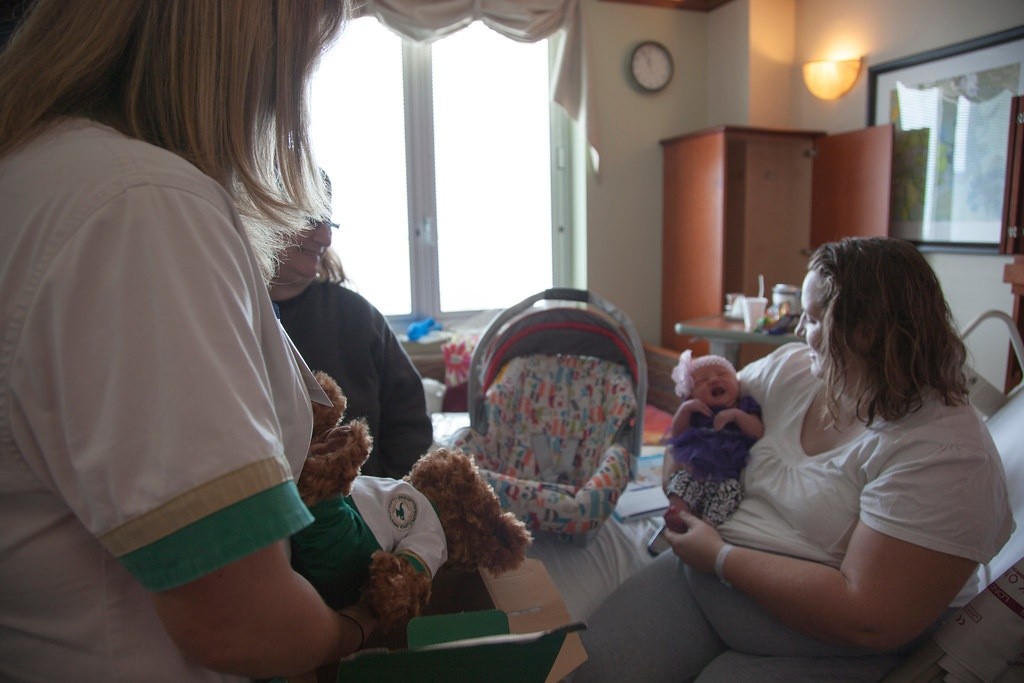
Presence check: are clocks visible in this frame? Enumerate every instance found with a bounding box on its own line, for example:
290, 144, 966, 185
628, 40, 674, 93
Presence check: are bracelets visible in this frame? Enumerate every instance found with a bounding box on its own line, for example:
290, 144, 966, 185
714, 544, 734, 587
341, 609, 370, 648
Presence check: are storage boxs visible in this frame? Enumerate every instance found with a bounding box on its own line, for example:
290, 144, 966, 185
318, 558, 590, 683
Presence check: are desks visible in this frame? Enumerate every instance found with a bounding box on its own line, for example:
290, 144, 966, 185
674, 314, 810, 374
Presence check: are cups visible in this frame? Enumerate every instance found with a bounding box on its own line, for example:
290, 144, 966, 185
772, 284, 801, 313
743, 297, 768, 334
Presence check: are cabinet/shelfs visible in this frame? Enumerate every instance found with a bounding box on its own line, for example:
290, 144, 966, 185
659, 122, 895, 375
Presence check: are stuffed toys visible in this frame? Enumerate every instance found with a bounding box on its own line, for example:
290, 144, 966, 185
290, 368, 536, 629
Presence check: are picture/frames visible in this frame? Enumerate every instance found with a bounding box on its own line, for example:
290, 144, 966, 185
865, 26, 1023, 256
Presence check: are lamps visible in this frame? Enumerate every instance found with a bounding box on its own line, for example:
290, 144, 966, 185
801, 56, 862, 101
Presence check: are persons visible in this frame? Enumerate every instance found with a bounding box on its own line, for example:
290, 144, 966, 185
265, 166, 434, 479
661, 349, 764, 533
0, 0, 372, 683
558, 235, 1017, 683
